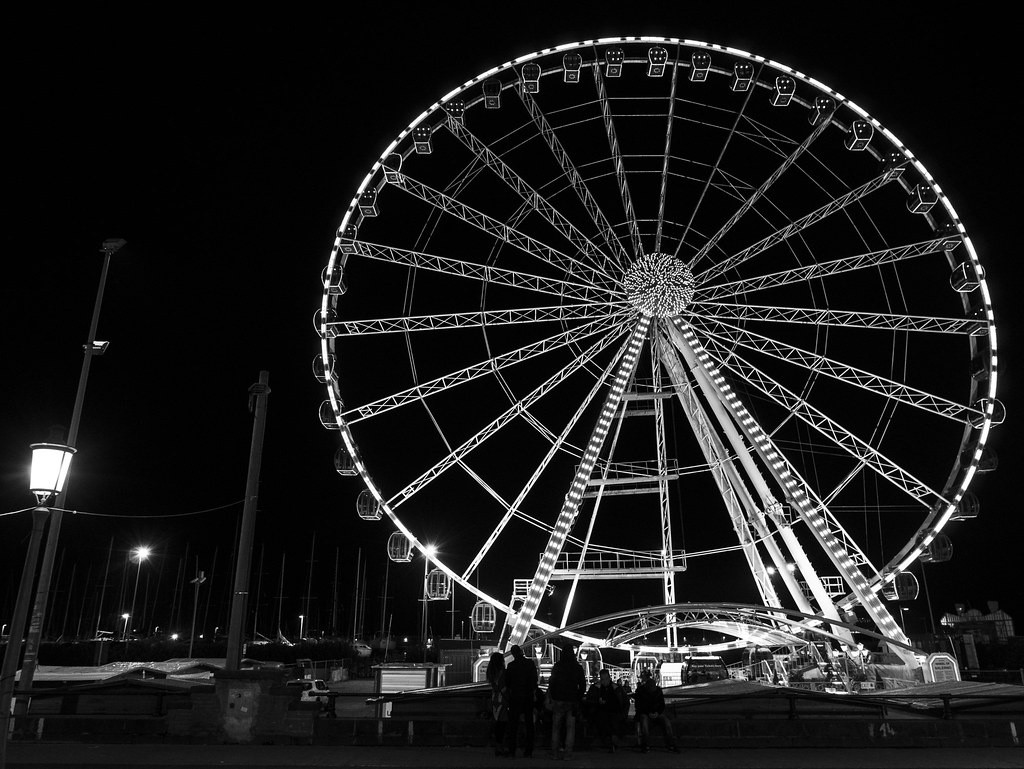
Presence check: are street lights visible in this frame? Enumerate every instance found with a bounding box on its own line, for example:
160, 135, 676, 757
0, 428, 77, 769
225, 382, 272, 670
119, 545, 152, 638
417, 542, 440, 656
189, 571, 207, 657
9, 237, 128, 731
121, 612, 130, 642
899, 607, 908, 636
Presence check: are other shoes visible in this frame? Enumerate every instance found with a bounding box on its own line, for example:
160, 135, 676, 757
495, 751, 504, 756
504, 751, 515, 756
609, 744, 615, 753
640, 746, 654, 754
524, 752, 532, 756
664, 746, 680, 754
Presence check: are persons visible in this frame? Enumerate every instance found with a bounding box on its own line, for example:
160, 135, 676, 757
615, 679, 644, 717
586, 669, 626, 753
549, 644, 586, 761
639, 679, 680, 753
486, 645, 538, 757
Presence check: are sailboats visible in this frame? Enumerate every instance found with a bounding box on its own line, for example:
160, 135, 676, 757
349, 549, 372, 658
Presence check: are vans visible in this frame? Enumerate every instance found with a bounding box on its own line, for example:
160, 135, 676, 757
285, 679, 330, 707
